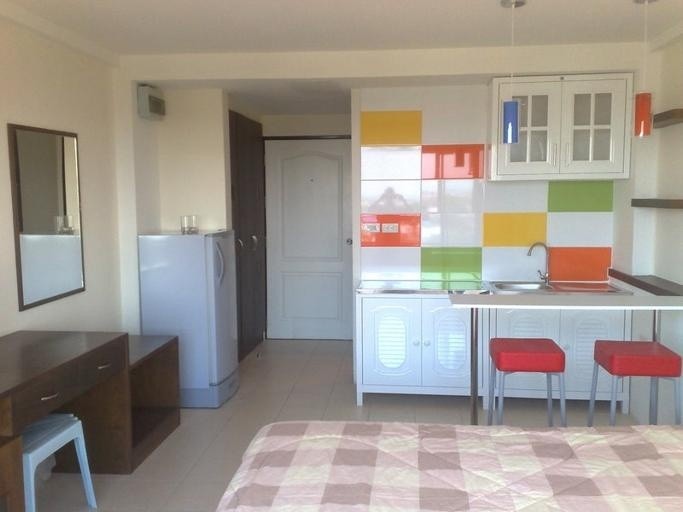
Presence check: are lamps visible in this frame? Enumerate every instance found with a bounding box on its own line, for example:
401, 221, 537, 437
502, 0, 520, 145
632, 1, 652, 138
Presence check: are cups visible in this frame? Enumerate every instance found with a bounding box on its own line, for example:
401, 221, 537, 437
54, 215, 75, 234
181, 215, 197, 236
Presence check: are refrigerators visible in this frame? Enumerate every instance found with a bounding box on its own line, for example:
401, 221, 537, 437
19, 234, 84, 305
137, 229, 241, 408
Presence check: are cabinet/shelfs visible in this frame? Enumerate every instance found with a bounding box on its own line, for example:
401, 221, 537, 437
355, 295, 488, 407
484, 72, 633, 183
490, 309, 632, 414
0, 330, 181, 512
608, 108, 682, 295
229, 112, 266, 362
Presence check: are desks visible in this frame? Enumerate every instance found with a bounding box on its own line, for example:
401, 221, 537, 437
451, 292, 682, 431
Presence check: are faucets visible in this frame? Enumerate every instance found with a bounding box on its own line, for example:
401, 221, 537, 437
528, 242, 549, 284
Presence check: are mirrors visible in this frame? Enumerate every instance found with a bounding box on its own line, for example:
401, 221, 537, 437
6, 124, 86, 313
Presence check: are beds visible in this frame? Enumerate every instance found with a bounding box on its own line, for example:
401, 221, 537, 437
216, 421, 683, 511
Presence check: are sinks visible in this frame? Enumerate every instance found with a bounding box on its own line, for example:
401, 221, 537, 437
488, 281, 556, 293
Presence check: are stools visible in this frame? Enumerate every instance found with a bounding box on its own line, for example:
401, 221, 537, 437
486, 337, 682, 429
21, 413, 97, 512
